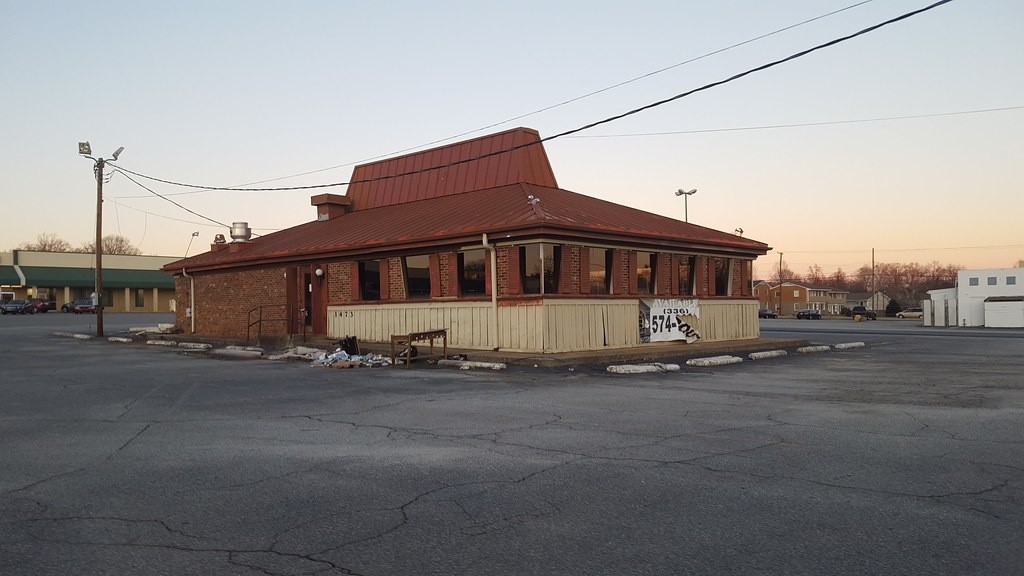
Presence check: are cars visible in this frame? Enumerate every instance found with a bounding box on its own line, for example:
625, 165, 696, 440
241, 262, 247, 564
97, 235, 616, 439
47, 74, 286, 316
75, 299, 103, 314
797, 309, 822, 320
758, 308, 778, 320
61, 300, 86, 313
0, 301, 8, 315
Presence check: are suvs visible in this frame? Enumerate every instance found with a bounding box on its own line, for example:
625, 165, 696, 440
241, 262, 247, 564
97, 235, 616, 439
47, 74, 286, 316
3, 300, 32, 315
852, 306, 877, 321
29, 298, 51, 313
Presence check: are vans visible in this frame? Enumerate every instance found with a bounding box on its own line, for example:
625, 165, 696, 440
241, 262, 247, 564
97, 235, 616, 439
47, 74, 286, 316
896, 307, 925, 319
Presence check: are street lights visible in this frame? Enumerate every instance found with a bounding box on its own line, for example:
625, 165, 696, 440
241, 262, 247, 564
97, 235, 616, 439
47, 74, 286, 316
675, 189, 698, 223
777, 251, 784, 315
78, 141, 124, 337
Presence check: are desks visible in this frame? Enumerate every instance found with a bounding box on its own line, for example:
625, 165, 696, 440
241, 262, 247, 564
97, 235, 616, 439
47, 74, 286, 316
391, 328, 449, 368
